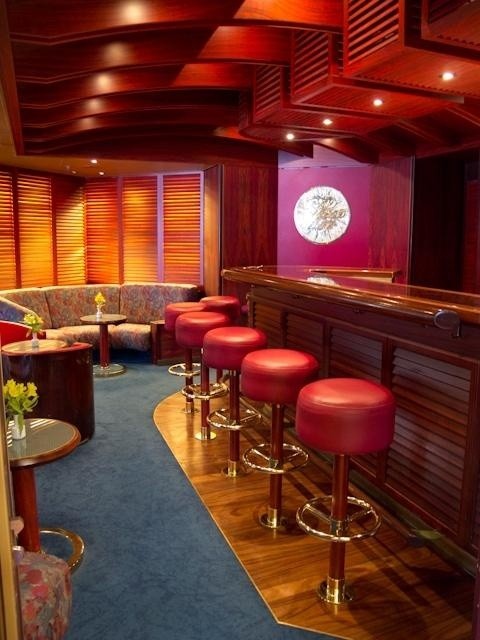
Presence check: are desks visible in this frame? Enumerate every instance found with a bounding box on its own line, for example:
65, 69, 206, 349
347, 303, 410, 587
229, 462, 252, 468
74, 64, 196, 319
80, 314, 128, 377
7, 418, 84, 574
0, 339, 93, 445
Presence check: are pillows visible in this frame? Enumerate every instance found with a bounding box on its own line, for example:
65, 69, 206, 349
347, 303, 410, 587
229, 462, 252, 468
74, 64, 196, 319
0, 283, 198, 329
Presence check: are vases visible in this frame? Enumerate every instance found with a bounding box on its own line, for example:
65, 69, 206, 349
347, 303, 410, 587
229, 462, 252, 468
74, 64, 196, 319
11, 415, 26, 440
31, 332, 39, 347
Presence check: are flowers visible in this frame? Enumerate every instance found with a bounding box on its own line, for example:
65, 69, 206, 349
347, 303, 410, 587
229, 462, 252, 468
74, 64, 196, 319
3, 379, 39, 436
18, 313, 44, 338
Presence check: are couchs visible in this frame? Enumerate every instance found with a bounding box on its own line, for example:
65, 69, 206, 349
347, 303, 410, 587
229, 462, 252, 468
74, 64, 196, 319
0, 283, 206, 366
